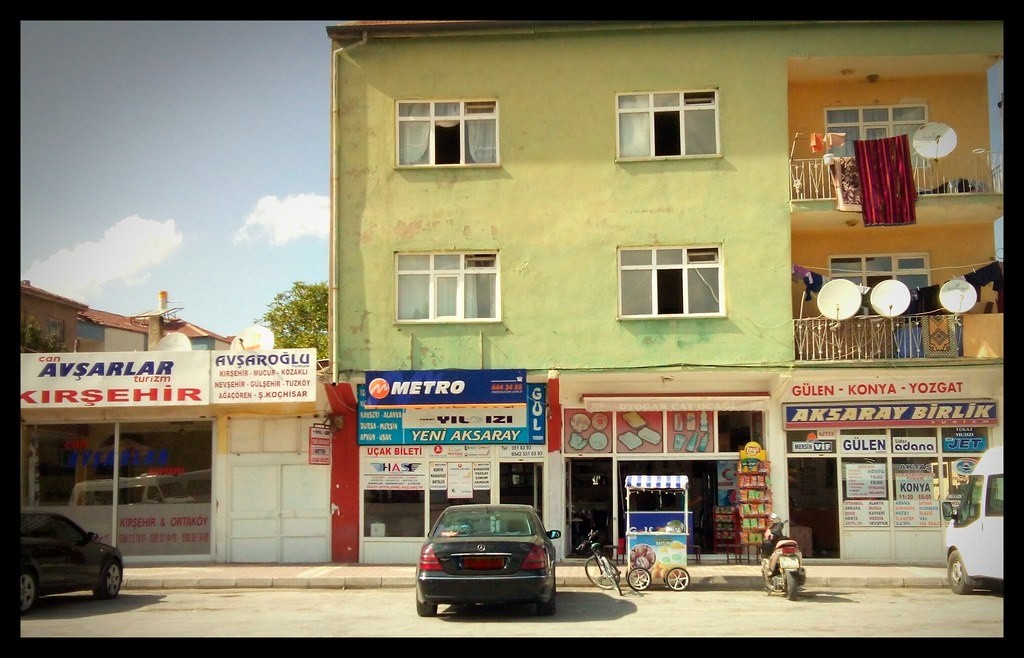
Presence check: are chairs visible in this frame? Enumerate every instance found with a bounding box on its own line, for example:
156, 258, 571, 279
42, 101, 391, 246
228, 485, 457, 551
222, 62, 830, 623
457, 519, 478, 534
725, 528, 750, 564
507, 519, 526, 534
687, 528, 701, 564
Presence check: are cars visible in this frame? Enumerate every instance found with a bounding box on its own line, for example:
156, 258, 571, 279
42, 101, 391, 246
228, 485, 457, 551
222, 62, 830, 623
415, 503, 561, 617
21, 508, 124, 617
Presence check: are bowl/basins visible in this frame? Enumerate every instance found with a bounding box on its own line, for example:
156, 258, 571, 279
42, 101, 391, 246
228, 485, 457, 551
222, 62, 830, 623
629, 544, 657, 572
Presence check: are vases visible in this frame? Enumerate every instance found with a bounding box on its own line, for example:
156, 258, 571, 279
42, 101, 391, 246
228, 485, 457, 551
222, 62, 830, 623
823, 153, 834, 166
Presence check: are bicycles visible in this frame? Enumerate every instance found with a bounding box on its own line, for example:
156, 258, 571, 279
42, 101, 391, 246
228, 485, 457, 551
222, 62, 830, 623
576, 529, 624, 598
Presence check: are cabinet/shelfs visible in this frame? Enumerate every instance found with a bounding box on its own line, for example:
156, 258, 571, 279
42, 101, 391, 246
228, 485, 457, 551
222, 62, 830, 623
712, 507, 739, 554
738, 471, 769, 548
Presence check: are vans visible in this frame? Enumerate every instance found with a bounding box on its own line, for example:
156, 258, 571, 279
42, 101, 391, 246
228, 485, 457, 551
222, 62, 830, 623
178, 469, 212, 503
942, 444, 1004, 594
68, 472, 197, 506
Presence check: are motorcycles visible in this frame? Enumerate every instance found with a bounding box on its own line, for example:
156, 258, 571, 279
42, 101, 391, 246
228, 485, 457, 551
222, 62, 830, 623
756, 512, 807, 601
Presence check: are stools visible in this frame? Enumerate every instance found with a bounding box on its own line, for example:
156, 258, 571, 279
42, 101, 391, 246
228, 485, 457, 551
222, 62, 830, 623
741, 543, 759, 565
604, 545, 624, 566
